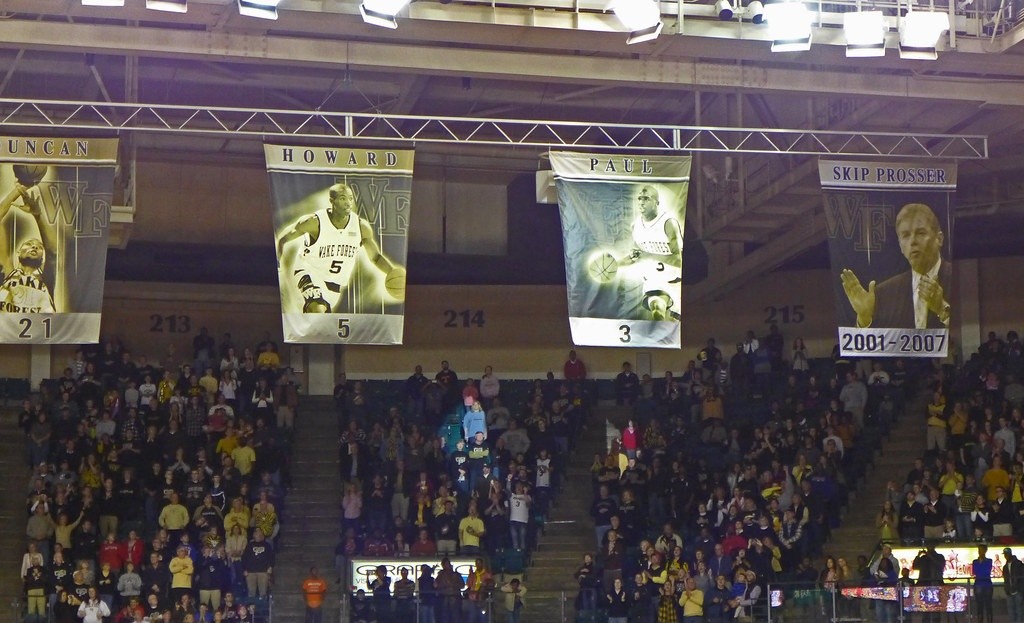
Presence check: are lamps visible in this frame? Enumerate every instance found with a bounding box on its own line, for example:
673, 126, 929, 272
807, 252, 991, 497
81, 0, 951, 62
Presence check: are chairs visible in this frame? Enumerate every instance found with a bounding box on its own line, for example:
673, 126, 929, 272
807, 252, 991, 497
344, 375, 689, 428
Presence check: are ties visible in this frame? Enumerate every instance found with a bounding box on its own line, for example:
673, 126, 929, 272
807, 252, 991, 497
916, 275, 927, 329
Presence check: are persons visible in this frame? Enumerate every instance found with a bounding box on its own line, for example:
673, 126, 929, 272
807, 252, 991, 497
1003, 549, 1024, 623
304, 567, 327, 623
971, 544, 994, 622
840, 203, 950, 329
587, 186, 682, 321
334, 350, 588, 621
18, 327, 302, 623
276, 185, 405, 315
0, 163, 60, 311
577, 325, 946, 623
939, 331, 1024, 546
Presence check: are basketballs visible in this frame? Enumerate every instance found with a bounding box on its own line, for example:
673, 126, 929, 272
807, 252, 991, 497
587, 252, 617, 282
12, 165, 48, 185
385, 269, 405, 300
